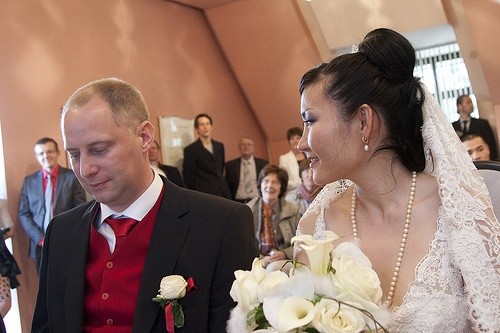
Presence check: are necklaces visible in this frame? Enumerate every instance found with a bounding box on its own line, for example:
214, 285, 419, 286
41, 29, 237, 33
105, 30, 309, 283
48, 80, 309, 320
350, 168, 417, 310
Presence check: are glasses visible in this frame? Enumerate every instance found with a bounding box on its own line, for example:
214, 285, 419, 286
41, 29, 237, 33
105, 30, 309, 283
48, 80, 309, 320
261, 178, 281, 186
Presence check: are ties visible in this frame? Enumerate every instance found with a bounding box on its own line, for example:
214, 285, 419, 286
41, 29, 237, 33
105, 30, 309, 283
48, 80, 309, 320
104, 217, 139, 250
463, 120, 467, 134
244, 161, 253, 195
43, 174, 53, 237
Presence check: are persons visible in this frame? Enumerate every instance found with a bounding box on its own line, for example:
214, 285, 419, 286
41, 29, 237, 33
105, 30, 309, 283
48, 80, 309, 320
227, 138, 270, 204
225, 28, 500, 333
278, 127, 307, 194
451, 94, 496, 162
0, 226, 21, 333
182, 113, 232, 200
246, 165, 303, 276
148, 141, 186, 189
285, 158, 326, 218
30, 78, 260, 333
18, 137, 87, 278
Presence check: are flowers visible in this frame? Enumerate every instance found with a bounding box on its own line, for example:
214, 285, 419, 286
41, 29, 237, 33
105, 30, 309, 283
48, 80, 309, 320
152, 274, 197, 333
229, 212, 383, 333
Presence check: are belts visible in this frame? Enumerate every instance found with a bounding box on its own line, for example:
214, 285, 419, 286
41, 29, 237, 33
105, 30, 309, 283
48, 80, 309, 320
234, 200, 250, 203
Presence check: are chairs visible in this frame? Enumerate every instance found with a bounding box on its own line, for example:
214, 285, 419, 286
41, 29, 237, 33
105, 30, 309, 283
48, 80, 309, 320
472, 160, 500, 225
